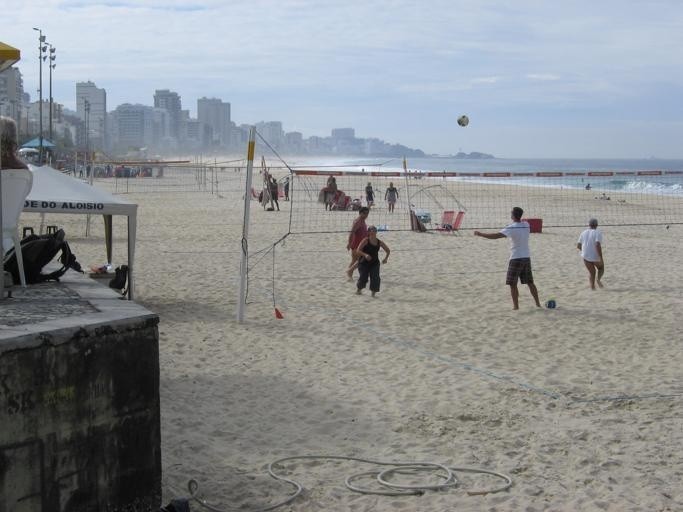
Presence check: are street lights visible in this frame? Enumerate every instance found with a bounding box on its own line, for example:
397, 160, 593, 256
81, 96, 91, 152
45, 42, 56, 162
32, 26, 46, 166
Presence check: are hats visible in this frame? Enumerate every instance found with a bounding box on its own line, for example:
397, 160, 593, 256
589, 219, 598, 225
368, 226, 377, 231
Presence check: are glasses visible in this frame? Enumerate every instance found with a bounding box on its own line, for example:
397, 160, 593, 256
363, 211, 369, 214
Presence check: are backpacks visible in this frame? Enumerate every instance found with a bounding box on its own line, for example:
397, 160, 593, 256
109, 266, 130, 296
4, 229, 73, 283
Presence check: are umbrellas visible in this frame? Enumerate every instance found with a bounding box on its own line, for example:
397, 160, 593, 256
21, 137, 57, 147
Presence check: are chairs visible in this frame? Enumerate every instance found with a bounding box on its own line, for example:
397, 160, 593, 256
0, 169, 33, 299
415, 208, 464, 237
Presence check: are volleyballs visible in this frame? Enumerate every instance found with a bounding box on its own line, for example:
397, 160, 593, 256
545, 297, 558, 308
457, 115, 469, 126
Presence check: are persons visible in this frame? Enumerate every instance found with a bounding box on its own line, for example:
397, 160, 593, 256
355, 225, 391, 297
474, 208, 541, 310
251, 174, 290, 211
0, 116, 28, 168
326, 177, 337, 211
366, 182, 375, 210
346, 206, 370, 281
15, 147, 148, 179
576, 219, 605, 290
344, 199, 361, 210
384, 181, 399, 213
327, 174, 334, 185
